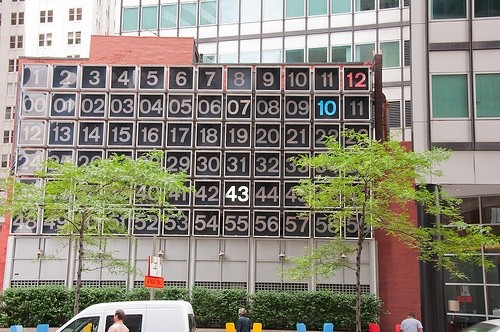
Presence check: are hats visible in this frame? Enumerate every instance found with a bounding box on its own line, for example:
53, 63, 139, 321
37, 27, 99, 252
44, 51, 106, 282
238, 308, 247, 315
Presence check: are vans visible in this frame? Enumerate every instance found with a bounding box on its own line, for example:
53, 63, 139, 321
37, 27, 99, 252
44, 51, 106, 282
53, 298, 197, 332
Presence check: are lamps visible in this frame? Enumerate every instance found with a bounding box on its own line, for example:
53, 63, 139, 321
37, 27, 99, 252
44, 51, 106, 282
158, 250, 165, 256
340, 252, 346, 259
99, 250, 104, 254
219, 251, 225, 256
36, 249, 43, 256
448, 300, 459, 324
279, 251, 285, 258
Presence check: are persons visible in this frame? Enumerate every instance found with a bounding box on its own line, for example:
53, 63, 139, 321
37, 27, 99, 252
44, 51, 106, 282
400, 313, 423, 332
236, 308, 250, 332
108, 309, 129, 332
